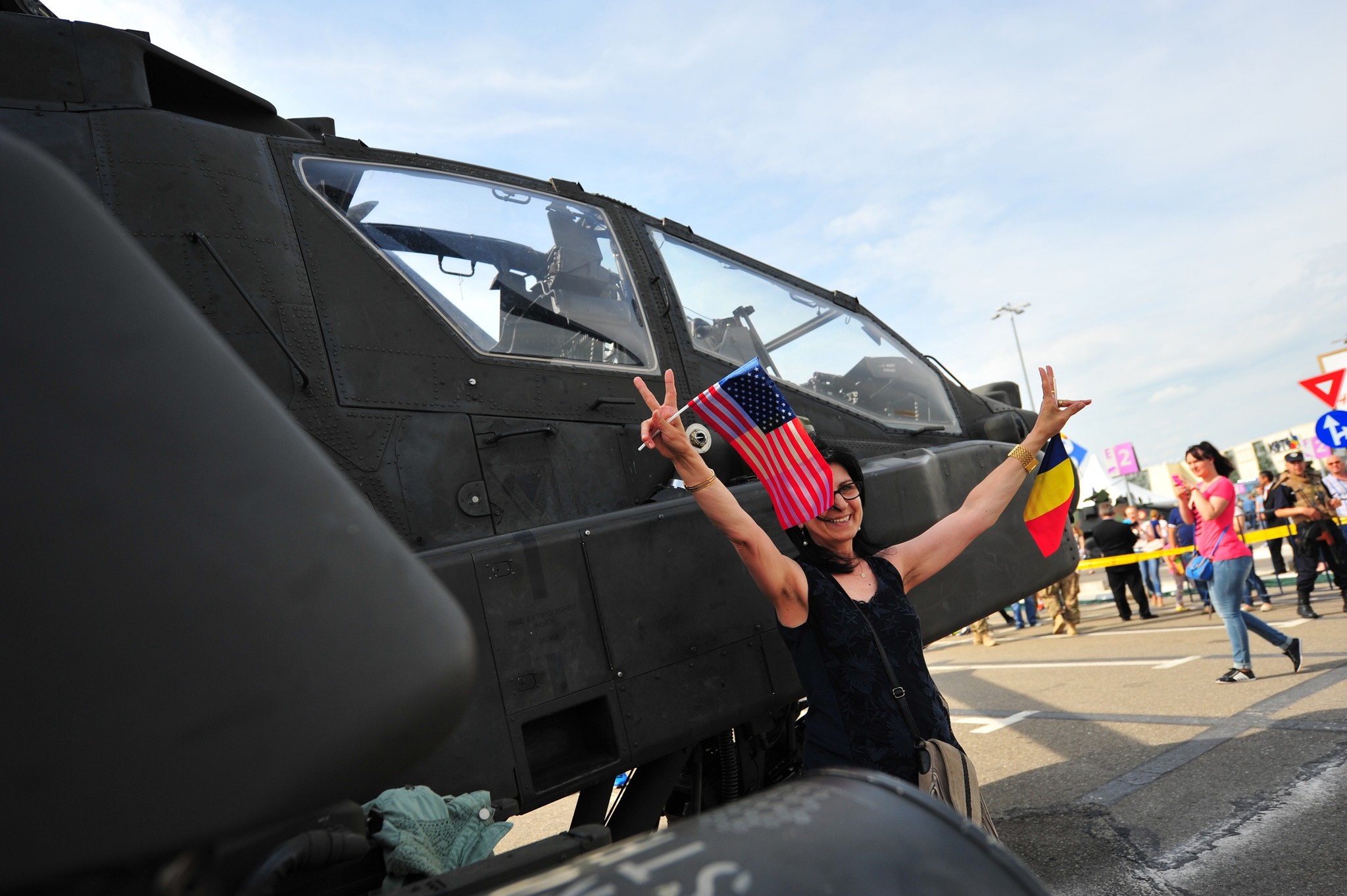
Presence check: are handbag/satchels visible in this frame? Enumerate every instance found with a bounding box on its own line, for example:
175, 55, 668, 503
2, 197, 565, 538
917, 738, 982, 830
1185, 556, 1213, 581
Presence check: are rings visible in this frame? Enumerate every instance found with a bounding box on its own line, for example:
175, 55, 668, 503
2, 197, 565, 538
1051, 390, 1055, 393
1058, 400, 1062, 407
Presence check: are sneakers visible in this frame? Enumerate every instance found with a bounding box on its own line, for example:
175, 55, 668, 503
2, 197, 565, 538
1282, 638, 1303, 672
1216, 668, 1257, 684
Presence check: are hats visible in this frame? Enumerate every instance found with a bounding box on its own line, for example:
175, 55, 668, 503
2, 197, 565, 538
1284, 452, 1303, 462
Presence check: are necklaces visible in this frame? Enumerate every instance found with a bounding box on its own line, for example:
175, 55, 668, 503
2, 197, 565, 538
847, 559, 866, 578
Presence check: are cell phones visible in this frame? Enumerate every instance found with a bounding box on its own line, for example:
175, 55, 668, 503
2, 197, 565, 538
1172, 474, 1185, 488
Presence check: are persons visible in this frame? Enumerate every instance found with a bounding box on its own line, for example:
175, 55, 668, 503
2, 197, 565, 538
630, 366, 1091, 822
1072, 502, 1272, 621
1174, 442, 1303, 684
1233, 452, 1347, 619
949, 568, 1082, 648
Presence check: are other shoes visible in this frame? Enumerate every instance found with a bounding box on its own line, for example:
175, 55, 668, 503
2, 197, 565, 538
1176, 605, 1190, 612
1052, 614, 1067, 634
973, 632, 997, 647
1122, 616, 1130, 620
1141, 610, 1158, 619
1297, 604, 1317, 617
1260, 603, 1273, 611
1202, 606, 1216, 614
1240, 603, 1256, 611
1066, 623, 1078, 634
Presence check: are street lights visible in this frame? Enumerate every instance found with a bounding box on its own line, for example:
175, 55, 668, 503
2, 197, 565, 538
992, 301, 1037, 413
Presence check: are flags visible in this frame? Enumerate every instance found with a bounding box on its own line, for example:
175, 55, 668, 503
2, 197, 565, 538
688, 358, 834, 531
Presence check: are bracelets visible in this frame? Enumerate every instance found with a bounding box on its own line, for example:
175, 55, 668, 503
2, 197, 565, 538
682, 468, 715, 493
1009, 443, 1039, 474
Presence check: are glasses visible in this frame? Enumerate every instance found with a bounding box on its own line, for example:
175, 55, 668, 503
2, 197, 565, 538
1329, 461, 1340, 465
834, 481, 862, 500
